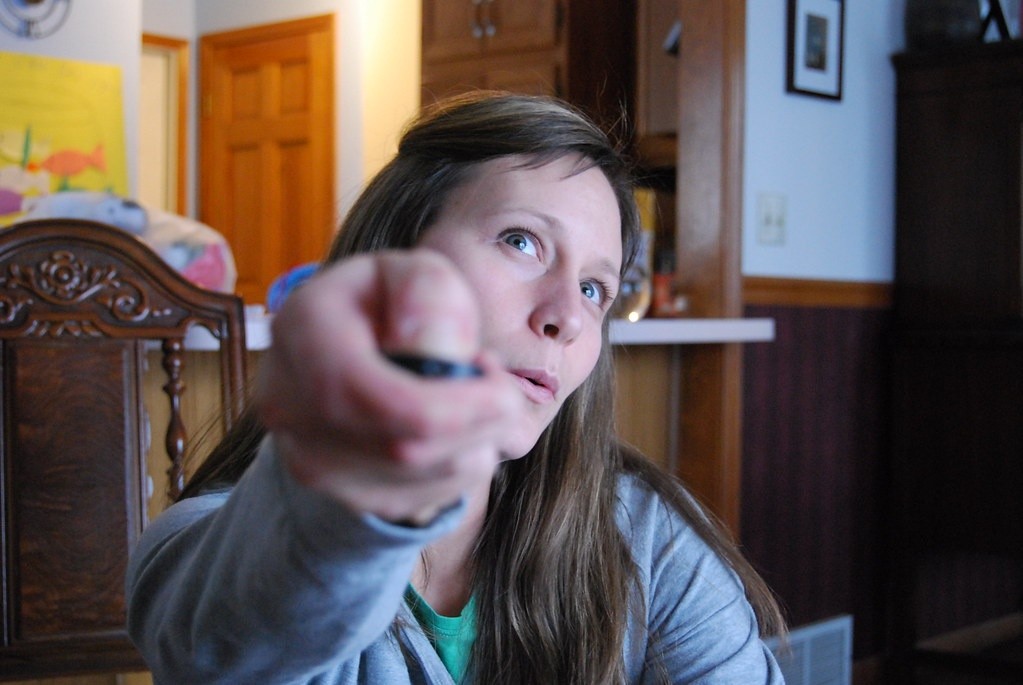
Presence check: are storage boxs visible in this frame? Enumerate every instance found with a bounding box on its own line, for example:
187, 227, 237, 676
617, 186, 676, 320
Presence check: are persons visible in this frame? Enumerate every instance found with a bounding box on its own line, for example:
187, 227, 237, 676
124, 92, 793, 685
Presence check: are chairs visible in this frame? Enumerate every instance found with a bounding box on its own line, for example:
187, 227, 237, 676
1, 215, 244, 684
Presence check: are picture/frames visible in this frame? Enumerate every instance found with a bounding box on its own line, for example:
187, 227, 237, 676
783, 0, 847, 100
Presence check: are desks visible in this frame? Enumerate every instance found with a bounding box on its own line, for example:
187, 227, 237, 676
0, 314, 780, 684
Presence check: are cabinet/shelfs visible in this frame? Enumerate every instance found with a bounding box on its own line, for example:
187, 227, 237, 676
420, 0, 682, 174
